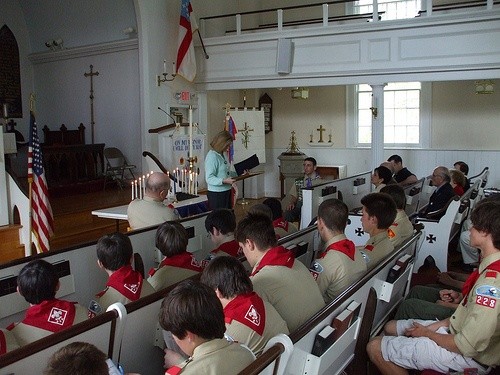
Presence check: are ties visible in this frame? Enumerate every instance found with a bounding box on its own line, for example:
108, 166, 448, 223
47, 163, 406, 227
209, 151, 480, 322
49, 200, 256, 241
461, 260, 500, 304
306, 179, 312, 187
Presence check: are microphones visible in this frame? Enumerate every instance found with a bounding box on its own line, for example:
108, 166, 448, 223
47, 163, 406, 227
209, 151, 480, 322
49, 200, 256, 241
158, 107, 176, 125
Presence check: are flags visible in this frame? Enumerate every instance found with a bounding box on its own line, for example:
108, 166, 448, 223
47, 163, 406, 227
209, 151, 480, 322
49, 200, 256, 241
25, 110, 56, 254
176, 0, 200, 82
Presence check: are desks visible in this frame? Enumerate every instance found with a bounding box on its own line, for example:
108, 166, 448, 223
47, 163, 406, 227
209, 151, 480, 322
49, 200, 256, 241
92, 195, 207, 234
316, 165, 348, 179
39, 143, 108, 180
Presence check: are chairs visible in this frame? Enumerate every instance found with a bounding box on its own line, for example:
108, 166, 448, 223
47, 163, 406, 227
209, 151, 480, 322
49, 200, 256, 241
104, 148, 137, 190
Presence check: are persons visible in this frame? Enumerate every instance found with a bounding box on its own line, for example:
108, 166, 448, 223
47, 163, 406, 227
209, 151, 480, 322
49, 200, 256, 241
204, 130, 248, 212
285, 157, 322, 222
126, 172, 182, 232
0, 154, 500, 375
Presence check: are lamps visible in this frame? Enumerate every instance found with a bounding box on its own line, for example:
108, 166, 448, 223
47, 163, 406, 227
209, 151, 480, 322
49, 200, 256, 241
291, 87, 310, 100
46, 38, 64, 50
157, 61, 176, 86
475, 80, 496, 95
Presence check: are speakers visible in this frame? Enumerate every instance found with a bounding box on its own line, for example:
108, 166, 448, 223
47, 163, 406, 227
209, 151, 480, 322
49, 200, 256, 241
276, 37, 291, 74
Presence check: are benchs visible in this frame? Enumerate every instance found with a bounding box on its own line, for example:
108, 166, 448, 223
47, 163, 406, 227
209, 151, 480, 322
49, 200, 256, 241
343, 179, 425, 247
298, 170, 372, 230
0, 210, 423, 375
412, 167, 490, 276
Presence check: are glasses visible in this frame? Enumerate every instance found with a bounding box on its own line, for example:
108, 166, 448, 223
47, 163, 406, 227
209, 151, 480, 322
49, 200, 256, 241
433, 173, 441, 177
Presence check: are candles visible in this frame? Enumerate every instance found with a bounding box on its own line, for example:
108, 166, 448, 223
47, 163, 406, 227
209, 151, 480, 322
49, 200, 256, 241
5, 103, 8, 118
132, 169, 198, 201
